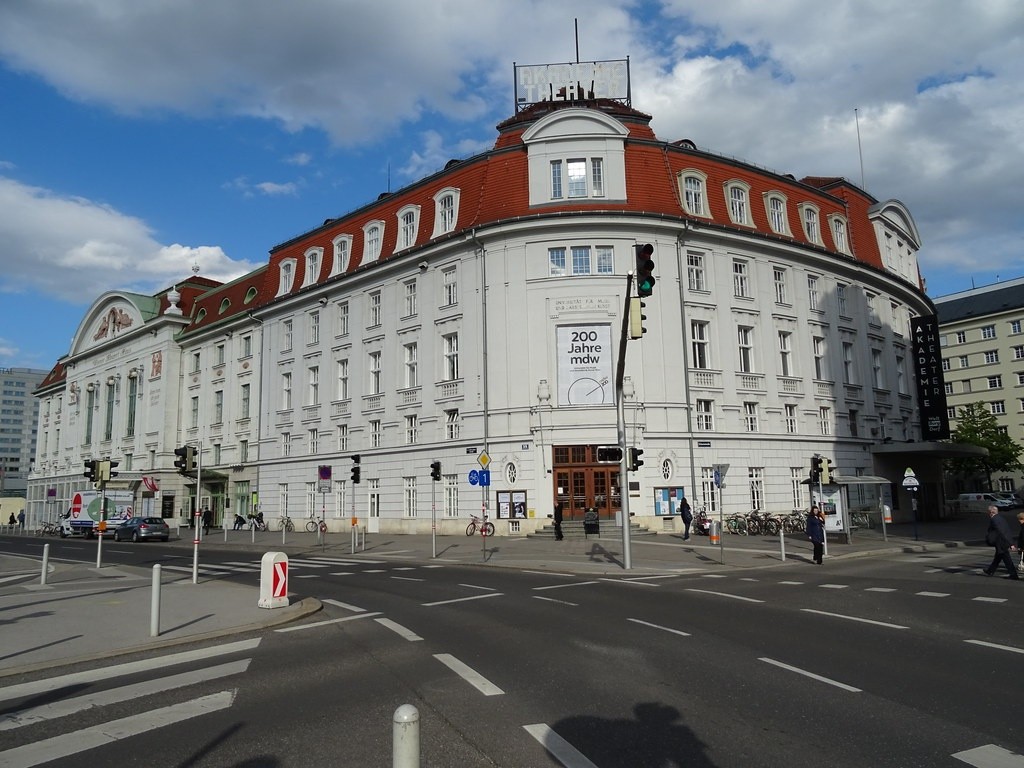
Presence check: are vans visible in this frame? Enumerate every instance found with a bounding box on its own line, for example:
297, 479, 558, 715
958, 492, 1014, 511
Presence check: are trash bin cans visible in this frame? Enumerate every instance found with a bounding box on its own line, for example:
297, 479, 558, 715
884, 505, 892, 523
710, 520, 720, 545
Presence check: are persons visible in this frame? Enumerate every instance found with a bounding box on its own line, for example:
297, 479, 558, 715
806, 506, 825, 565
553, 499, 563, 541
680, 497, 692, 542
232, 514, 246, 530
17, 510, 25, 528
202, 507, 212, 535
8, 513, 17, 529
1017, 512, 1024, 570
983, 506, 1018, 579
514, 503, 525, 517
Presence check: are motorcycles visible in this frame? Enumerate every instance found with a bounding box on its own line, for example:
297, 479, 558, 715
694, 511, 712, 536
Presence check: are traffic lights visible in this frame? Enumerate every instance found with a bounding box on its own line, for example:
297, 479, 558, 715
596, 446, 623, 463
628, 448, 644, 471
351, 454, 360, 463
629, 297, 647, 340
635, 243, 656, 297
811, 458, 834, 483
84, 461, 119, 482
430, 462, 441, 480
173, 447, 197, 470
351, 466, 360, 483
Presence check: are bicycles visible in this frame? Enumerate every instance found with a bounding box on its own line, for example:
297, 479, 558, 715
466, 514, 495, 537
726, 509, 874, 536
35, 520, 61, 537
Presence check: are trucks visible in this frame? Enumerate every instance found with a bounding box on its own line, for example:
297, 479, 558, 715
59, 490, 134, 539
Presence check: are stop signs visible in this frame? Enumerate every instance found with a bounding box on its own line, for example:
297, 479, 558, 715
72, 494, 82, 518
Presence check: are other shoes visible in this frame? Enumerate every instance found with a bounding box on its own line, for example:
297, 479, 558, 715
685, 538, 690, 541
821, 563, 824, 565
983, 568, 995, 575
1008, 574, 1018, 579
812, 556, 817, 563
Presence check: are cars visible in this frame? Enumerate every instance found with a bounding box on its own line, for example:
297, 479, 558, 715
114, 516, 170, 543
999, 492, 1023, 508
